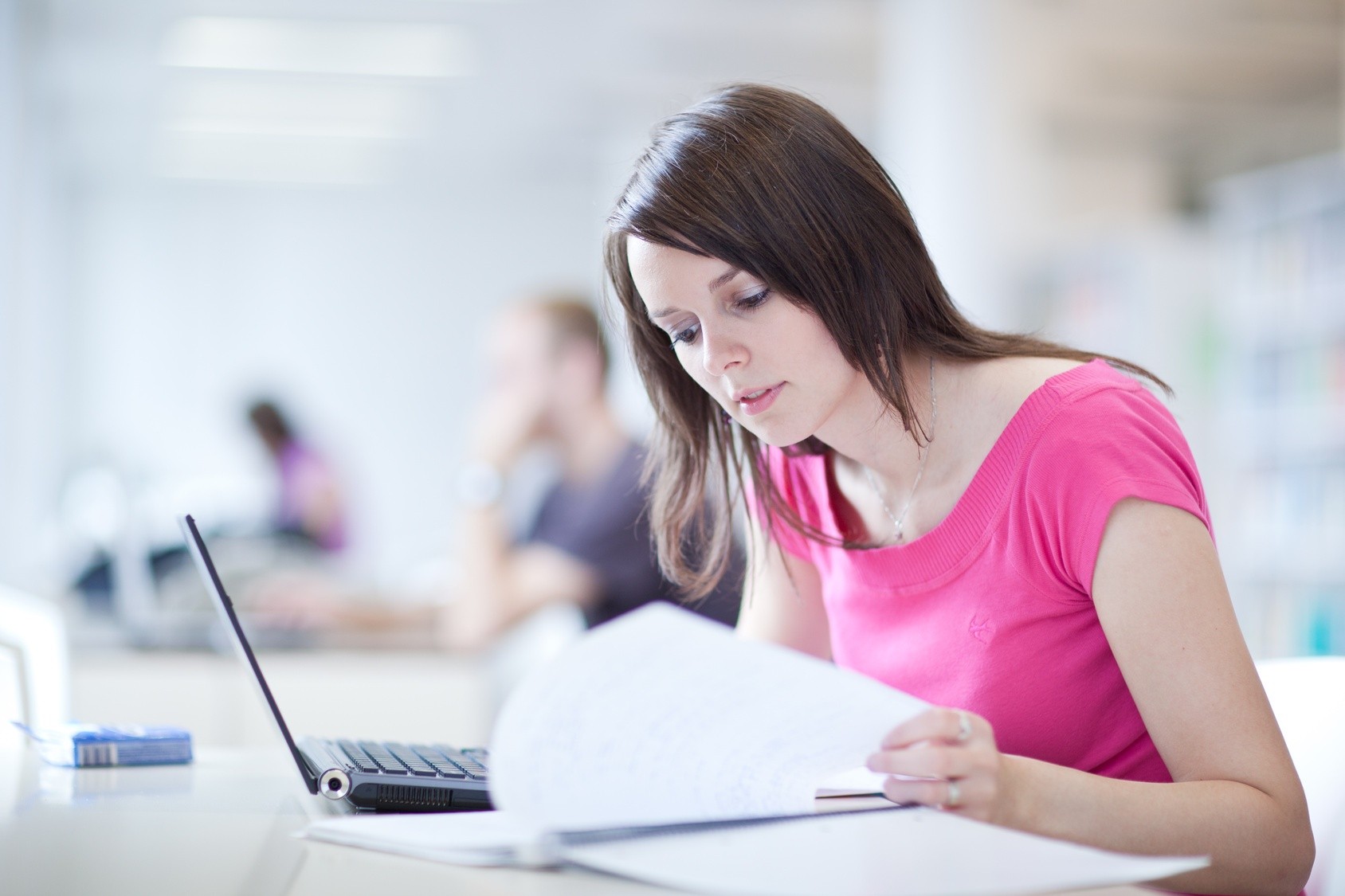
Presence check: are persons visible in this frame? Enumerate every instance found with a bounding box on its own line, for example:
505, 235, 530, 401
251, 400, 352, 556
446, 286, 747, 654
604, 83, 1316, 895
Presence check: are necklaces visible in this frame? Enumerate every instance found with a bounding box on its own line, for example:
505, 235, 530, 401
861, 352, 932, 540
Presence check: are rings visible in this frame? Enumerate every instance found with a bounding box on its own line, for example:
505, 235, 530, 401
950, 708, 972, 743
944, 783, 963, 809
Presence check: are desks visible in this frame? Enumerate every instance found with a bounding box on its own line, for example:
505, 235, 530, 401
0, 657, 1170, 896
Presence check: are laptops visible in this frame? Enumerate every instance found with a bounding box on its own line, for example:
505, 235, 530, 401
178, 513, 494, 815
